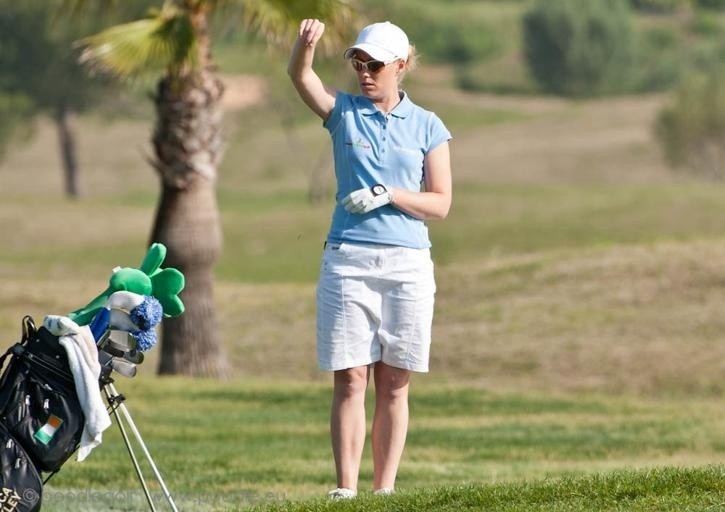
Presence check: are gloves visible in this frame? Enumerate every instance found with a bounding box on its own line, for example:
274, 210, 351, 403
340, 184, 391, 214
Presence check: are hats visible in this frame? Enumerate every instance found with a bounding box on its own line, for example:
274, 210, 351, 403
345, 21, 410, 62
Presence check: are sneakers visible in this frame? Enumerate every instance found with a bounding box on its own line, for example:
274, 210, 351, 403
370, 489, 395, 497
327, 488, 356, 502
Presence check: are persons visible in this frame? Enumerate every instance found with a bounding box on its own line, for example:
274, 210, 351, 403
283, 16, 453, 503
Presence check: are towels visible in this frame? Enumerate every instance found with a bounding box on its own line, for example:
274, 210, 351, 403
58, 324, 112, 463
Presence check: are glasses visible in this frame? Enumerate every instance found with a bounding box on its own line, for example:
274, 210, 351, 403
350, 56, 386, 70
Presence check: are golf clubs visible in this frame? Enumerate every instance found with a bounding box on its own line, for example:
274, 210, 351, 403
97, 330, 144, 378
